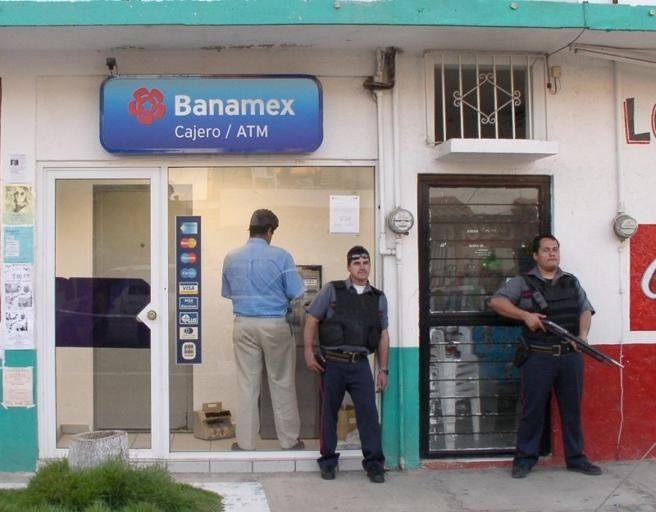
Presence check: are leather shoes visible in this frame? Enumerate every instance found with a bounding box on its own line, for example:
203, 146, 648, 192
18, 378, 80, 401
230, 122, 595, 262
367, 469, 385, 484
567, 461, 602, 476
321, 466, 336, 481
510, 462, 533, 478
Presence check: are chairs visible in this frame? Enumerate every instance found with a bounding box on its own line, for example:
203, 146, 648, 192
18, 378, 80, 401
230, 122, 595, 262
429, 326, 482, 450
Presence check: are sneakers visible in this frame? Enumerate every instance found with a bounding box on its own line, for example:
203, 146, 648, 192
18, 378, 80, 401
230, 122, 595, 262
281, 439, 305, 450
231, 442, 257, 451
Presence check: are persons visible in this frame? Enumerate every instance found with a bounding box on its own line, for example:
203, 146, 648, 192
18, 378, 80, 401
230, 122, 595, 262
487, 234, 603, 478
222, 208, 307, 451
474, 298, 523, 451
303, 245, 391, 481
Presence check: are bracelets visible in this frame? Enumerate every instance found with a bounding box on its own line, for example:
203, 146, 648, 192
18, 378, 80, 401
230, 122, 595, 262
379, 366, 389, 375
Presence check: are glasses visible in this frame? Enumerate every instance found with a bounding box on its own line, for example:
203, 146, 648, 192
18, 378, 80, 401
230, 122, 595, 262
351, 252, 370, 259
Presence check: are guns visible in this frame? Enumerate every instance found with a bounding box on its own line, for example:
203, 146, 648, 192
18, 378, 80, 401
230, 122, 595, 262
541, 320, 626, 370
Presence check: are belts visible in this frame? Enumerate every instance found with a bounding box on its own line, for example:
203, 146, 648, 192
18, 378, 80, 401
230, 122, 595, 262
323, 349, 368, 364
528, 342, 574, 357
